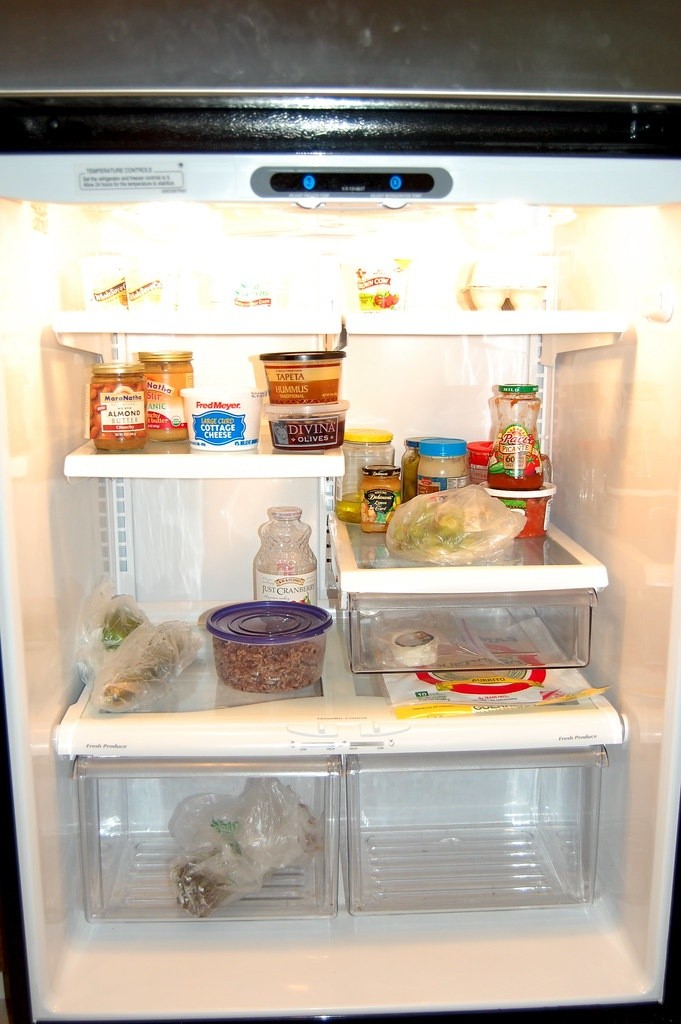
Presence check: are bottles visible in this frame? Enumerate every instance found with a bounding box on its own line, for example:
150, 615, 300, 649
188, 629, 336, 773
336, 431, 396, 523
253, 508, 318, 611
487, 383, 545, 488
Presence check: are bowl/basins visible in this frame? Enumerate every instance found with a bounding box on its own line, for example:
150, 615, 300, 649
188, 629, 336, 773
479, 482, 556, 539
206, 601, 334, 693
261, 399, 349, 451
179, 387, 267, 451
256, 351, 348, 409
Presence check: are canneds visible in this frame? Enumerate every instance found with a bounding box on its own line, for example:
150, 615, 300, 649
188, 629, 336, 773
89, 351, 194, 451
360, 465, 401, 533
401, 437, 469, 502
487, 383, 545, 489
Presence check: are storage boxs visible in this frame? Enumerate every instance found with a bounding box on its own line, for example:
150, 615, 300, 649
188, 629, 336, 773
327, 525, 599, 674
74, 744, 341, 923
340, 743, 611, 918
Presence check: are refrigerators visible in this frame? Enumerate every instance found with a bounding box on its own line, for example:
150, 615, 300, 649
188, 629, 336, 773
0, 0, 681, 1024
